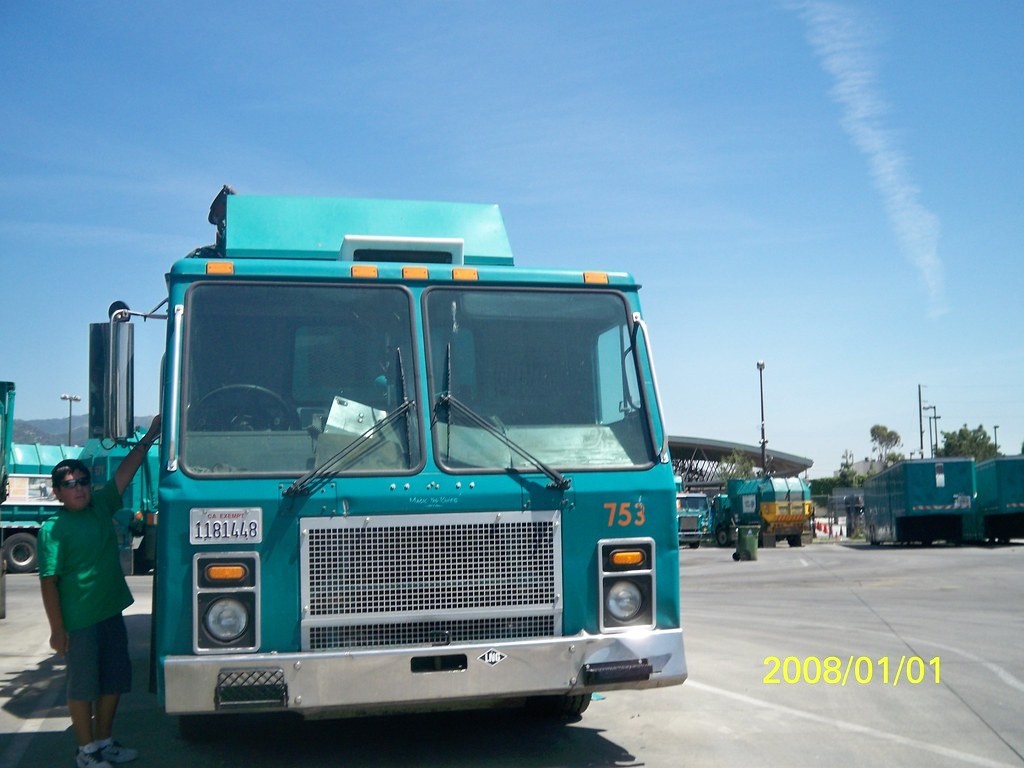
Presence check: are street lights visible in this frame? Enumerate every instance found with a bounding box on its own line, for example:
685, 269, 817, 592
928, 416, 941, 459
59, 393, 82, 445
922, 404, 938, 457
993, 424, 1000, 456
756, 360, 769, 476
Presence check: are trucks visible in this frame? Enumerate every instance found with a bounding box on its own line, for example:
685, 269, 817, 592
710, 471, 815, 549
1, 182, 815, 740
858, 452, 1023, 549
673, 475, 712, 551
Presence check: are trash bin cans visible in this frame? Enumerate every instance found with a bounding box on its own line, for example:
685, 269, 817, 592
730, 525, 762, 562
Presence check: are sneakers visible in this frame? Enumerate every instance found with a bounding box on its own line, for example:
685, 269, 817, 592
74, 748, 112, 768
98, 741, 139, 762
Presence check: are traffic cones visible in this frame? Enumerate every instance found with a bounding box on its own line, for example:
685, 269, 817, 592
815, 521, 840, 541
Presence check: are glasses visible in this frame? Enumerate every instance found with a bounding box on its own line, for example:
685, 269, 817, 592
60, 476, 90, 489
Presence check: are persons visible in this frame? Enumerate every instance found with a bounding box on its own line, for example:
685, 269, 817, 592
39, 413, 162, 768
